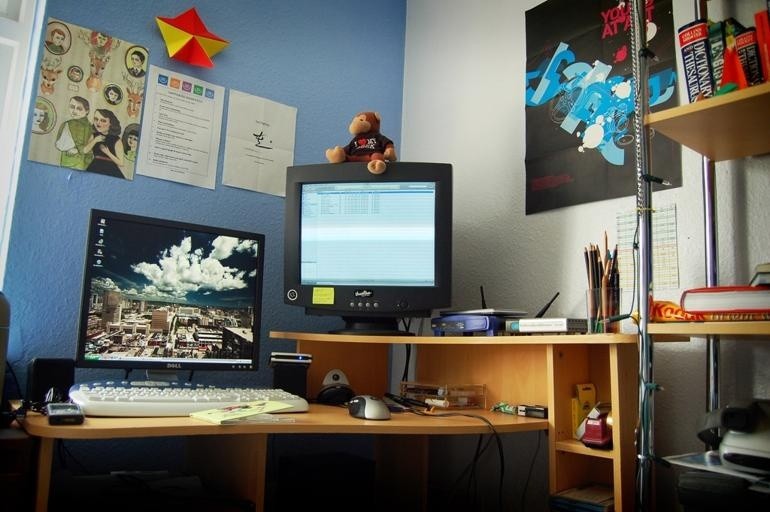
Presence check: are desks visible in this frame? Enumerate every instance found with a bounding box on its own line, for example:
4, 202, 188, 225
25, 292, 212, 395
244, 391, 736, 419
7, 335, 690, 511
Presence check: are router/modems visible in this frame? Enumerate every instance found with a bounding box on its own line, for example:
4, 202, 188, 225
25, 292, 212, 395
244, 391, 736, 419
267, 352, 312, 368
505, 317, 587, 334
431, 285, 559, 337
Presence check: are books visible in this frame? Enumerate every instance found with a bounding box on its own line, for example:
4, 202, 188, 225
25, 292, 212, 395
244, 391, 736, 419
677, 11, 770, 105
680, 261, 770, 315
190, 400, 297, 426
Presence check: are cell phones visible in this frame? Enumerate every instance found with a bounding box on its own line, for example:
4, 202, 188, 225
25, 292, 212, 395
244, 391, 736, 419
47, 403, 83, 425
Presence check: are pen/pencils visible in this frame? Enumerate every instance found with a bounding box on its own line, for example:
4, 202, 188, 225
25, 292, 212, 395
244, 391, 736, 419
581, 231, 621, 333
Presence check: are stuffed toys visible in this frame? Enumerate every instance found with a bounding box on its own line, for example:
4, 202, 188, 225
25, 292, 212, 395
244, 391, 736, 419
324, 111, 398, 176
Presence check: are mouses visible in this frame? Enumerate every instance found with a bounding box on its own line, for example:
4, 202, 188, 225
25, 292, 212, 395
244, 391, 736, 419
348, 394, 391, 421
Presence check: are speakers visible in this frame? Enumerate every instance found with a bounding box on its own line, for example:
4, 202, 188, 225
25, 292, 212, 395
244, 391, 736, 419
26, 358, 75, 402
273, 366, 306, 398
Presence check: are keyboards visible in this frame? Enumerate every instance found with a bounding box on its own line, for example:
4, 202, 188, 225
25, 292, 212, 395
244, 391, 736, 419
69, 379, 310, 418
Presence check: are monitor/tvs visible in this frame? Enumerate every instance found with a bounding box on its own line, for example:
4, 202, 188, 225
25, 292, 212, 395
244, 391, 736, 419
74, 207, 265, 371
283, 162, 452, 335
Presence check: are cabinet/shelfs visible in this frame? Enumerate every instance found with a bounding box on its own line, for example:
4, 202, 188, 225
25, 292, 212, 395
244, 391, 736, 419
631, 0, 769, 512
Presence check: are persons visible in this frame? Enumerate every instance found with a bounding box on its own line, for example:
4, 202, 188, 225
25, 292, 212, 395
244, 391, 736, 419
83, 109, 125, 178
95, 31, 110, 49
29, 103, 53, 133
126, 50, 145, 75
105, 86, 122, 103
47, 30, 66, 53
56, 96, 92, 170
126, 130, 136, 162
